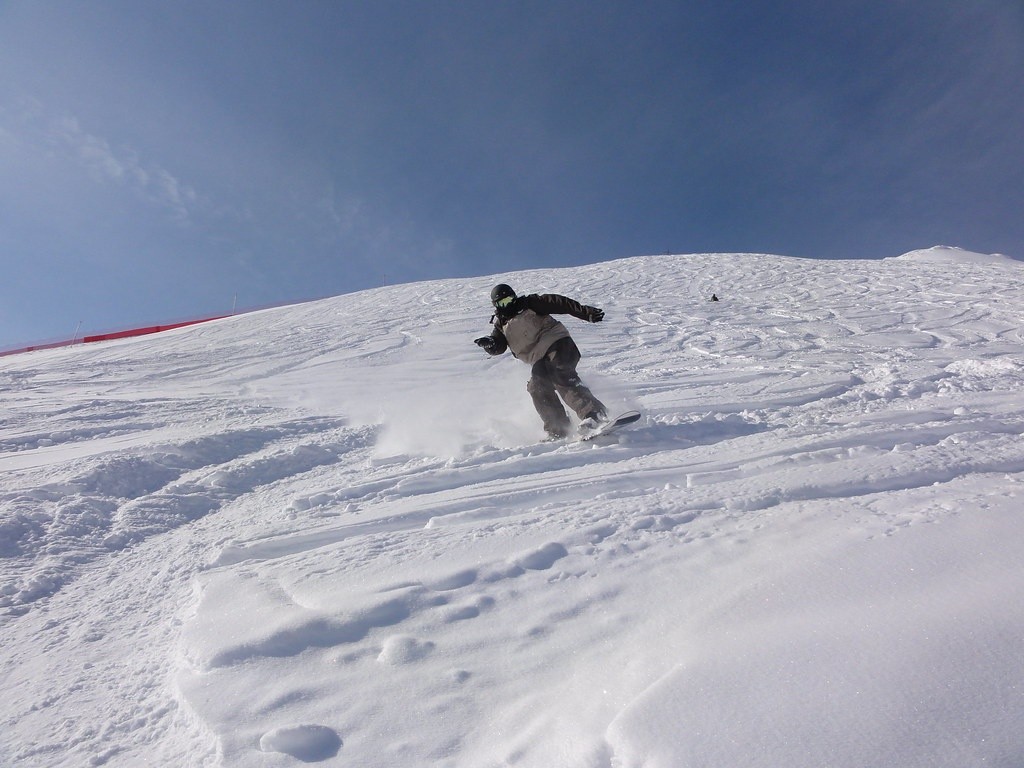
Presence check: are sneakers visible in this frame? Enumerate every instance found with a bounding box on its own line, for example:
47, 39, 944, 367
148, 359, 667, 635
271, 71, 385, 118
545, 425, 571, 438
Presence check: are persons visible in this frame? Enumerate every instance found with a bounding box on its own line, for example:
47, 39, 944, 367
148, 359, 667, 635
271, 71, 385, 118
474, 284, 606, 441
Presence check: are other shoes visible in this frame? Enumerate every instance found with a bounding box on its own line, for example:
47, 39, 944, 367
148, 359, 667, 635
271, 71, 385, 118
577, 406, 609, 435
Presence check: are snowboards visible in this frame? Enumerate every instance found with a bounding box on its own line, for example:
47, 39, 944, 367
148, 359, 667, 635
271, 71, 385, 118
536, 409, 643, 443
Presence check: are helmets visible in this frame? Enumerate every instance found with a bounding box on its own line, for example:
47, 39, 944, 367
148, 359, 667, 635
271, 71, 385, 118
491, 284, 516, 307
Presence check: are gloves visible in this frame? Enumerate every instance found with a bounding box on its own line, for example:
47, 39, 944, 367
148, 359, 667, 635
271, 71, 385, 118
586, 306, 605, 324
475, 336, 495, 350
